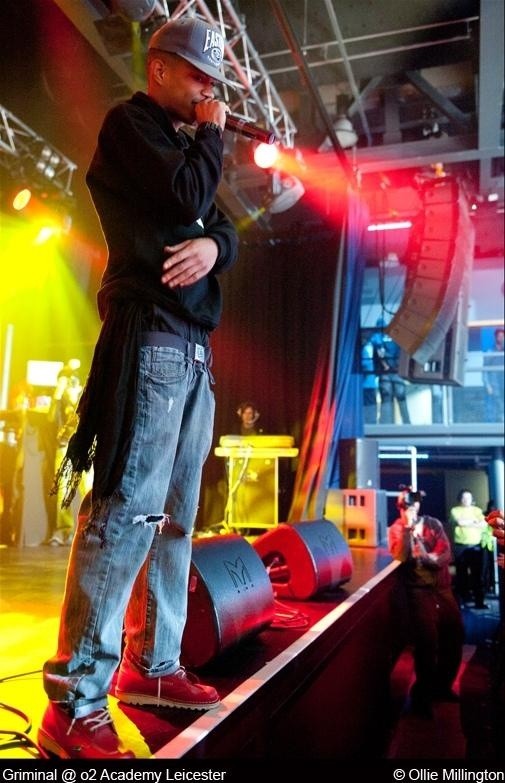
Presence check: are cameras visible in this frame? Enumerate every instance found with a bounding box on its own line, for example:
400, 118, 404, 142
398, 483, 426, 511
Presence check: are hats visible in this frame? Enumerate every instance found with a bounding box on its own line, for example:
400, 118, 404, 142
145, 16, 240, 92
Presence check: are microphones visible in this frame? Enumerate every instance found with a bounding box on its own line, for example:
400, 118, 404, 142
224, 112, 276, 145
248, 417, 258, 424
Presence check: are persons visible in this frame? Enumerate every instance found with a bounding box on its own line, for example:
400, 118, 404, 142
493, 327, 505, 351
489, 508, 505, 546
230, 400, 267, 434
37, 16, 278, 758
450, 488, 489, 609
496, 552, 505, 570
481, 499, 496, 592
388, 486, 465, 719
45, 366, 94, 546
373, 331, 412, 423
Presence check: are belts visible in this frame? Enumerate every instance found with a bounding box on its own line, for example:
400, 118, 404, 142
136, 327, 213, 368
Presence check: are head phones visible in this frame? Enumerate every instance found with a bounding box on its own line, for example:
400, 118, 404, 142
236, 401, 260, 417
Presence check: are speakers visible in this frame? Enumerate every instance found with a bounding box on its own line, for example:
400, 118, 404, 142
251, 519, 353, 601
384, 174, 476, 389
179, 532, 276, 668
340, 437, 381, 489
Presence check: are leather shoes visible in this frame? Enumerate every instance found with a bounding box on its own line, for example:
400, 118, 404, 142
35, 693, 136, 759
114, 656, 221, 711
